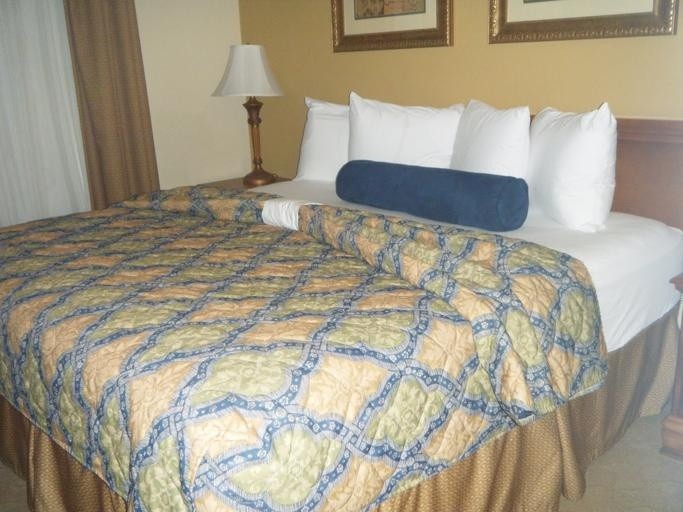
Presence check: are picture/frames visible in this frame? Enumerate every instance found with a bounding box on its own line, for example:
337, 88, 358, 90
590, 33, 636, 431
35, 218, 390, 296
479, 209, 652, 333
331, 1, 453, 52
488, 0, 679, 44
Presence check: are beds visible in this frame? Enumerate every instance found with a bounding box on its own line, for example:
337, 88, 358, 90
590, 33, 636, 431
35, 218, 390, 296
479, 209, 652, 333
1, 117, 683, 510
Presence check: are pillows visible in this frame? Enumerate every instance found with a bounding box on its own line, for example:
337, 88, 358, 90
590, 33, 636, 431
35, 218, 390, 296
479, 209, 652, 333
335, 160, 529, 232
448, 97, 530, 184
347, 90, 464, 169
291, 97, 349, 182
530, 101, 618, 231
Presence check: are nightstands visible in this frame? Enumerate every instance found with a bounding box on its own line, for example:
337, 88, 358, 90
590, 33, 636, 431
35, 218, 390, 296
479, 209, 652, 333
660, 276, 682, 459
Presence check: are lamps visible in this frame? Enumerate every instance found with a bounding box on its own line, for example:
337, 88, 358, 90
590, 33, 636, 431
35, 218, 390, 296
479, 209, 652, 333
209, 44, 285, 187
